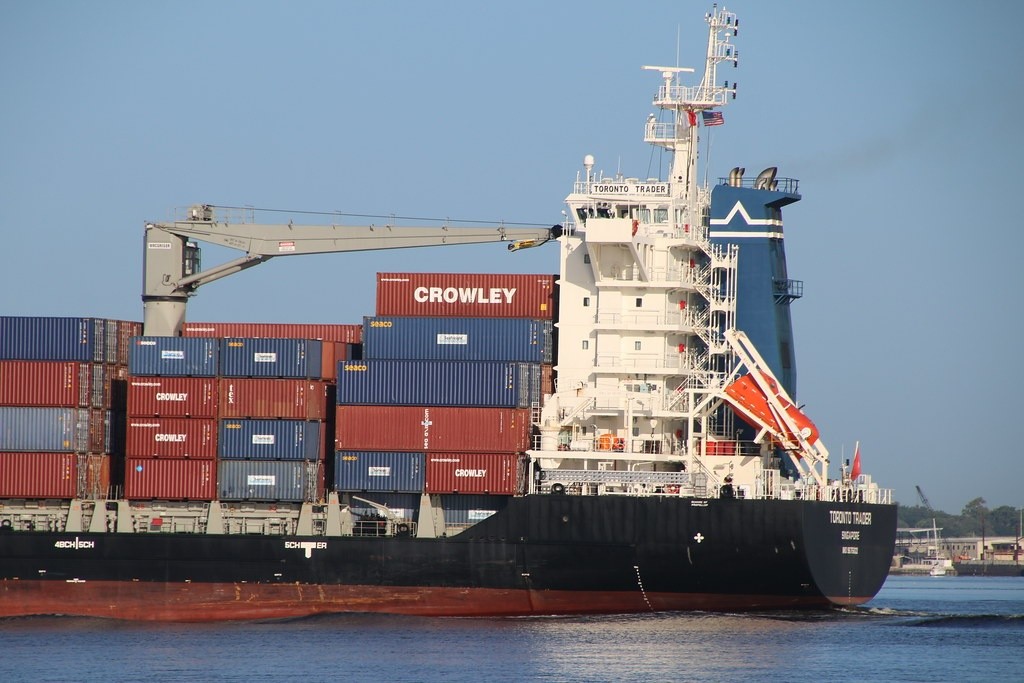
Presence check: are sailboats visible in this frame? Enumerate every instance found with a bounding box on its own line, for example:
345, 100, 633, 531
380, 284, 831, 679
928, 519, 946, 577
952, 500, 1024, 577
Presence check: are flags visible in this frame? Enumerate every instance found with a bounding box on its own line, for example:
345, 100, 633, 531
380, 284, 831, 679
850, 441, 862, 482
703, 111, 724, 126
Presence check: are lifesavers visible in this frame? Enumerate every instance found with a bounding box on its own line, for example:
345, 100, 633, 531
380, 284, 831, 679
550, 482, 566, 496
719, 492, 732, 503
396, 523, 410, 536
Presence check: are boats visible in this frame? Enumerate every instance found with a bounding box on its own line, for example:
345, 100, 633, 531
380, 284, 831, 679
0, 0, 901, 627
